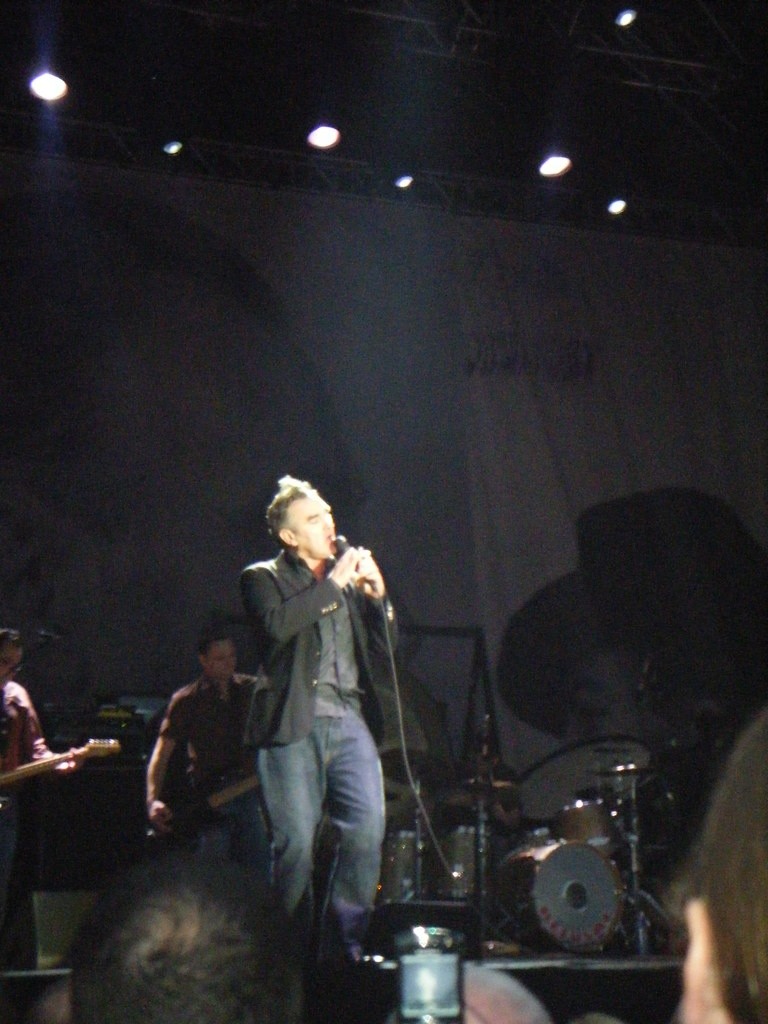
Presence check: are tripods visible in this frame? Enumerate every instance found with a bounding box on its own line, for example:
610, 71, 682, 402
613, 778, 674, 957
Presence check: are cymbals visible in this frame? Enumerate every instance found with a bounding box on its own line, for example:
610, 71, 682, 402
514, 738, 651, 820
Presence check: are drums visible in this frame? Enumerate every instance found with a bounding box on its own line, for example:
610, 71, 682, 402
548, 797, 621, 855
375, 831, 423, 902
494, 840, 628, 953
429, 825, 504, 905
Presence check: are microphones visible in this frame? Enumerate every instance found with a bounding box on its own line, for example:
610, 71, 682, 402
334, 536, 379, 593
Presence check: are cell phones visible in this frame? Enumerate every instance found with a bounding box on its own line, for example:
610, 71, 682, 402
399, 929, 465, 1024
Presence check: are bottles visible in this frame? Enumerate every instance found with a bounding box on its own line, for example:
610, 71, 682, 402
634, 913, 648, 954
394, 930, 462, 1024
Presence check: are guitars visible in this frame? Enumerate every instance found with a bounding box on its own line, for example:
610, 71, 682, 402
127, 768, 263, 857
0, 738, 120, 811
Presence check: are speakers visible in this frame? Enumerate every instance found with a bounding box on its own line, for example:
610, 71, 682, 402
11, 891, 96, 968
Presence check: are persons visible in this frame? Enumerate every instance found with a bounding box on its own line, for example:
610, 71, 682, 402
670, 710, 768, 1024
430, 740, 520, 861
32, 859, 305, 1023
0, 628, 87, 929
238, 474, 404, 988
146, 626, 271, 888
